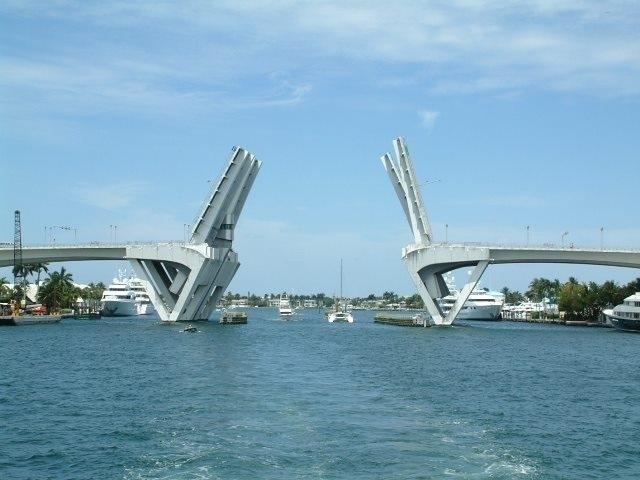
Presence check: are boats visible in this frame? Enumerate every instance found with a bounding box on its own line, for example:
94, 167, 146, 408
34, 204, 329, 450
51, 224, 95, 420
0, 315, 62, 326
100, 268, 154, 316
184, 328, 197, 332
439, 269, 505, 320
326, 299, 434, 327
601, 292, 640, 332
218, 311, 248, 324
279, 300, 294, 315
